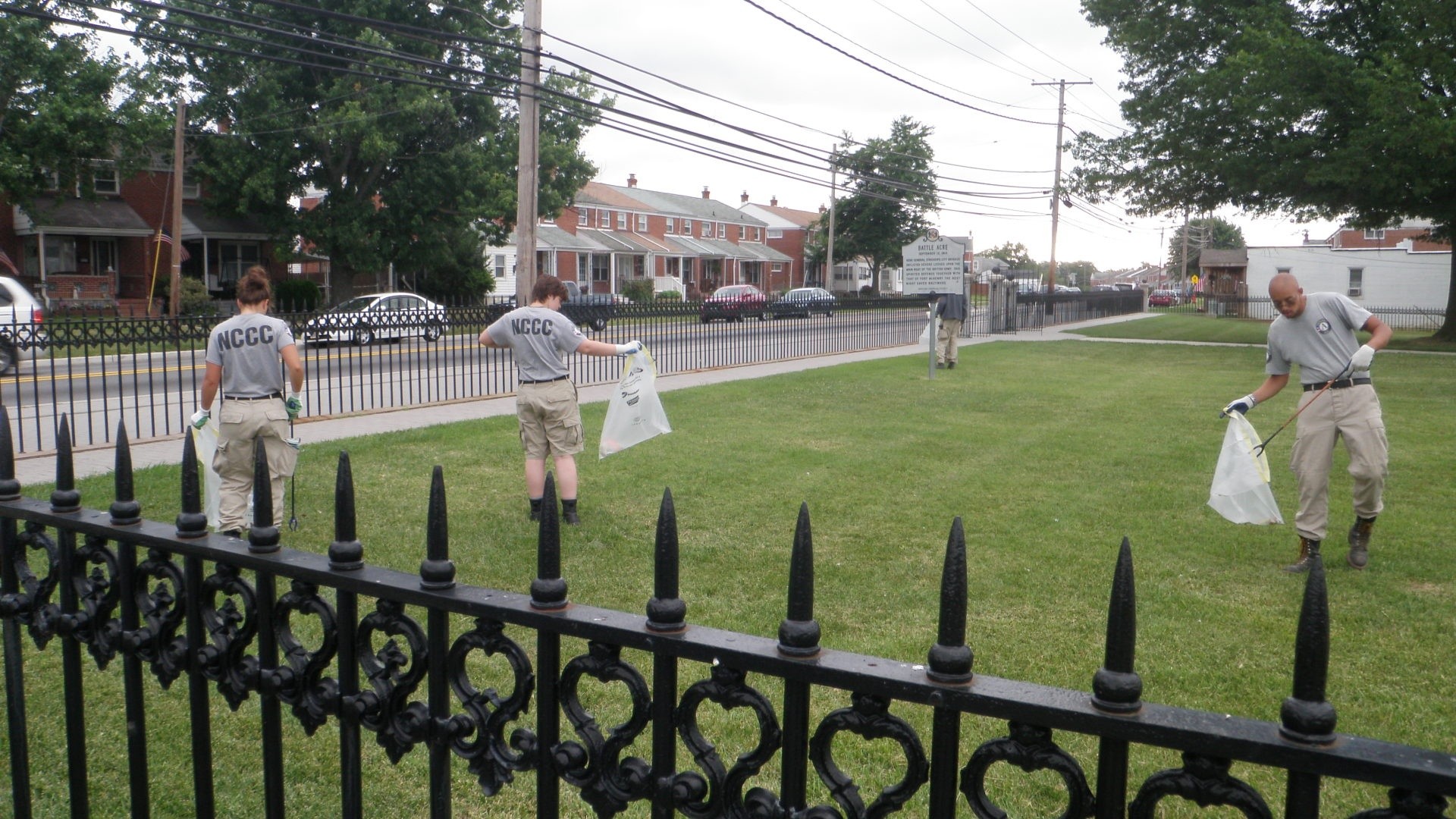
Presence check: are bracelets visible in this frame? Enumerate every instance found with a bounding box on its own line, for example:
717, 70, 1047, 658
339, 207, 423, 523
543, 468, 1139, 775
1247, 394, 1257, 407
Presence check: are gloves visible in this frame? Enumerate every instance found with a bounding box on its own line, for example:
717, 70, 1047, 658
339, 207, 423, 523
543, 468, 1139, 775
615, 340, 642, 354
1220, 396, 1254, 418
190, 405, 210, 430
285, 390, 302, 421
1347, 344, 1375, 378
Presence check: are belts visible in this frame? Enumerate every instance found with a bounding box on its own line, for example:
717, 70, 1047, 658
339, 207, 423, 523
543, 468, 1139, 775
225, 391, 280, 401
1304, 378, 1371, 391
520, 373, 569, 384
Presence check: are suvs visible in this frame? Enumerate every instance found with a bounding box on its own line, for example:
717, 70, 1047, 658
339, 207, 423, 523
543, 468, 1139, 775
0, 276, 51, 376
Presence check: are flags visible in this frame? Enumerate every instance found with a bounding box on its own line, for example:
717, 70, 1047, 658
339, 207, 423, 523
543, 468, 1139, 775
0, 249, 19, 276
153, 223, 191, 263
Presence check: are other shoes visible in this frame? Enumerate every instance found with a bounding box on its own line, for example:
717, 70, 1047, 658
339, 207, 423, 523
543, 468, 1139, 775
935, 363, 945, 368
530, 511, 541, 522
948, 362, 956, 370
562, 511, 580, 526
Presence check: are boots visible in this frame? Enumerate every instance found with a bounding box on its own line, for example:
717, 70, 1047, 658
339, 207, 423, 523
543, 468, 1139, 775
1283, 534, 1322, 574
1347, 516, 1376, 570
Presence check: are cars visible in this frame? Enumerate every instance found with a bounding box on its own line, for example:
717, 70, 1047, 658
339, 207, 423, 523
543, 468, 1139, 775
1009, 277, 1181, 309
771, 287, 838, 318
700, 284, 768, 323
299, 292, 446, 348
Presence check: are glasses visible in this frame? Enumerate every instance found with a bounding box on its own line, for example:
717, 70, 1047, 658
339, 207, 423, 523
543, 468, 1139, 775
1274, 295, 1296, 308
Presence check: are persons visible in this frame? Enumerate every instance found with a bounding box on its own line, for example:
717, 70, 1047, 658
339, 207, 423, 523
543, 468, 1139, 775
479, 274, 644, 523
936, 295, 968, 370
191, 267, 303, 543
1219, 274, 1392, 574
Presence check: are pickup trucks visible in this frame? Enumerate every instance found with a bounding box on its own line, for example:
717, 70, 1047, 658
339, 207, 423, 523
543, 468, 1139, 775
489, 280, 615, 332
